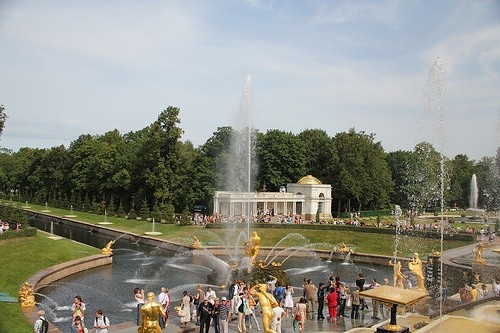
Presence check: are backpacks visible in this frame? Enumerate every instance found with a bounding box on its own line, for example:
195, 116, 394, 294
39, 318, 48, 333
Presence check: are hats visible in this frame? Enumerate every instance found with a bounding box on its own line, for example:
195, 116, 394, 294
222, 296, 226, 300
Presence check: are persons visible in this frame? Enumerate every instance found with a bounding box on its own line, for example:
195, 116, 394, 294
470, 284, 478, 302
334, 212, 365, 226
194, 206, 304, 225
179, 279, 260, 333
389, 253, 425, 288
475, 243, 483, 259
34, 310, 49, 333
139, 292, 165, 333
244, 232, 261, 263
159, 287, 170, 328
94, 310, 109, 333
372, 220, 426, 231
266, 273, 392, 333
250, 284, 278, 333
467, 226, 485, 237
134, 288, 144, 326
403, 277, 411, 290
71, 296, 86, 333
480, 281, 500, 302
0, 219, 22, 230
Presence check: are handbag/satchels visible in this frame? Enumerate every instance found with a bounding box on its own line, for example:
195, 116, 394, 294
239, 303, 243, 311
178, 309, 186, 316
296, 313, 301, 320
